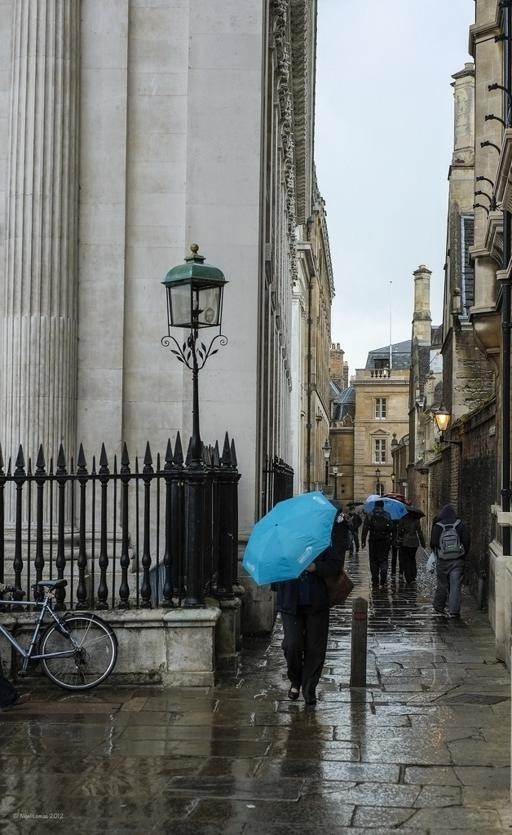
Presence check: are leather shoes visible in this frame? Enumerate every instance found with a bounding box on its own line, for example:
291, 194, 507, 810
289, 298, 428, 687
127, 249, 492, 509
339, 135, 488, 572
303, 689, 318, 706
289, 685, 299, 701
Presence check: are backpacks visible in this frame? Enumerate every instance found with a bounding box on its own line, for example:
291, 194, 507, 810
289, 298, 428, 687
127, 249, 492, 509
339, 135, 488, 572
435, 519, 466, 559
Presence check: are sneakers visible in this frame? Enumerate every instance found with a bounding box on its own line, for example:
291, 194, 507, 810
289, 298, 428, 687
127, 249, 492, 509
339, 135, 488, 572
430, 607, 463, 621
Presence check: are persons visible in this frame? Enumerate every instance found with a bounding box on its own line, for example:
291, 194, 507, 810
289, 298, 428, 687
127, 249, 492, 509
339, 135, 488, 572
270, 550, 346, 704
330, 501, 471, 619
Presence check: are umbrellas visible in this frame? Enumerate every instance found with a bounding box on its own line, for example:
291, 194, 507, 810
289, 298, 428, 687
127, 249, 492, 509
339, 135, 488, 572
242, 491, 338, 586
383, 493, 412, 506
363, 495, 408, 520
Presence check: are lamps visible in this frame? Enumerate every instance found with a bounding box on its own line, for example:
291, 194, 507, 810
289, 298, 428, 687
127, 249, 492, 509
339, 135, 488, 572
427, 399, 463, 454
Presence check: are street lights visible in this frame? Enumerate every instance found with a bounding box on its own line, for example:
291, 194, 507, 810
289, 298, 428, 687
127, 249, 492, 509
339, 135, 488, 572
391, 473, 396, 492
332, 465, 339, 500
160, 242, 231, 614
322, 437, 331, 486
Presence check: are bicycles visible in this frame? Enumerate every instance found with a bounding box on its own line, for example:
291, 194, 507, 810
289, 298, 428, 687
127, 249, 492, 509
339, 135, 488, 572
0, 574, 119, 694
